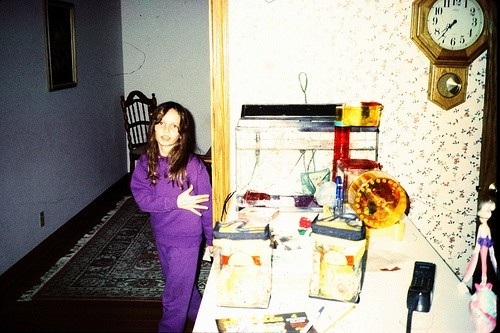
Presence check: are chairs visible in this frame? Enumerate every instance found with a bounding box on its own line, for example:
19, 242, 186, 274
119, 90, 158, 173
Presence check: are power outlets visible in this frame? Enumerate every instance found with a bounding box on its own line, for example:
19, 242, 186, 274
40, 211, 44, 227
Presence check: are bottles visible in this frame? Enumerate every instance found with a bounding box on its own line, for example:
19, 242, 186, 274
335, 176, 344, 214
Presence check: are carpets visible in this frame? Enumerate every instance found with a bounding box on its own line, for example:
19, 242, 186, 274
16, 195, 212, 305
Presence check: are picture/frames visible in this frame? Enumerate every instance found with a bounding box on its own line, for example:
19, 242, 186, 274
41, 0, 79, 93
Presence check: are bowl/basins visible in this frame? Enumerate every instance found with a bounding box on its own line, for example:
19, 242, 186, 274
336, 105, 383, 126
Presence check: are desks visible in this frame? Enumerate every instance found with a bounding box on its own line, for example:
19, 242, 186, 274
188, 191, 473, 333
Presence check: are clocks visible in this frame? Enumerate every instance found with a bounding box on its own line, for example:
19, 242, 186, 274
409, 0, 491, 111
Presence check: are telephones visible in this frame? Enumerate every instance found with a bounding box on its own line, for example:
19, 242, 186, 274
406, 261, 436, 312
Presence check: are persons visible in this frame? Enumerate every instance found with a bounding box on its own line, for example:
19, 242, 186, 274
131, 100, 214, 333
